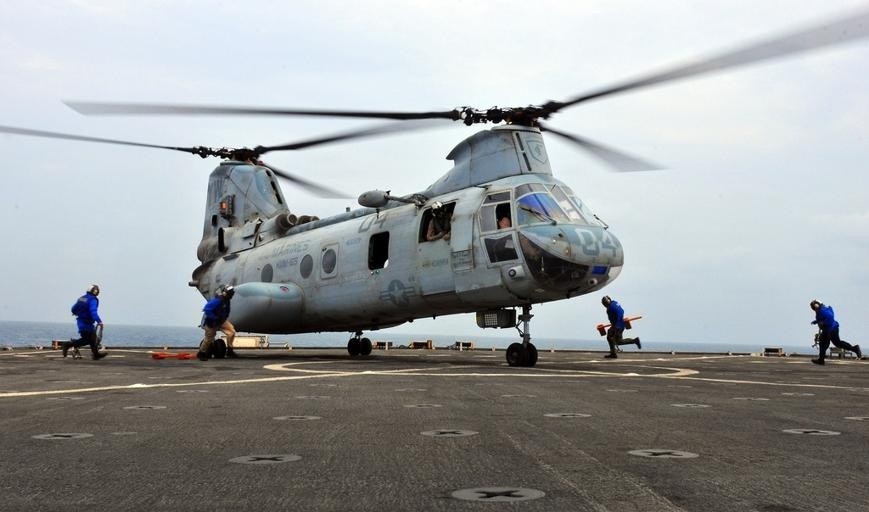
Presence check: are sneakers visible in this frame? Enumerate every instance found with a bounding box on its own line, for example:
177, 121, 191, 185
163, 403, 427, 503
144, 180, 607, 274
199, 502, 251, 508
634, 337, 641, 349
604, 353, 617, 358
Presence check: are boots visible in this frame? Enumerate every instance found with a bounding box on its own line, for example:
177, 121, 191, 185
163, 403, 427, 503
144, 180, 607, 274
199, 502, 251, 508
226, 347, 236, 358
851, 345, 861, 359
811, 356, 826, 365
61, 342, 73, 357
197, 351, 208, 361
90, 348, 107, 360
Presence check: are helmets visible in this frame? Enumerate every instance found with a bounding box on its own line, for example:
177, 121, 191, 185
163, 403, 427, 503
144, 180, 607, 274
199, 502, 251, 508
222, 285, 234, 296
602, 296, 611, 306
810, 299, 822, 310
87, 285, 98, 297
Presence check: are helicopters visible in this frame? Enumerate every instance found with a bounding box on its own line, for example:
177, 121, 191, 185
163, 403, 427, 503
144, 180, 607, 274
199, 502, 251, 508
1, 5, 869, 369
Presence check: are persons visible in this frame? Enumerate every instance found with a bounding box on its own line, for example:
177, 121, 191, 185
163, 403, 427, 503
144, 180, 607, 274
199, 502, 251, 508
601, 295, 642, 359
499, 215, 511, 230
426, 201, 453, 242
808, 299, 861, 366
195, 285, 240, 360
61, 284, 108, 361
